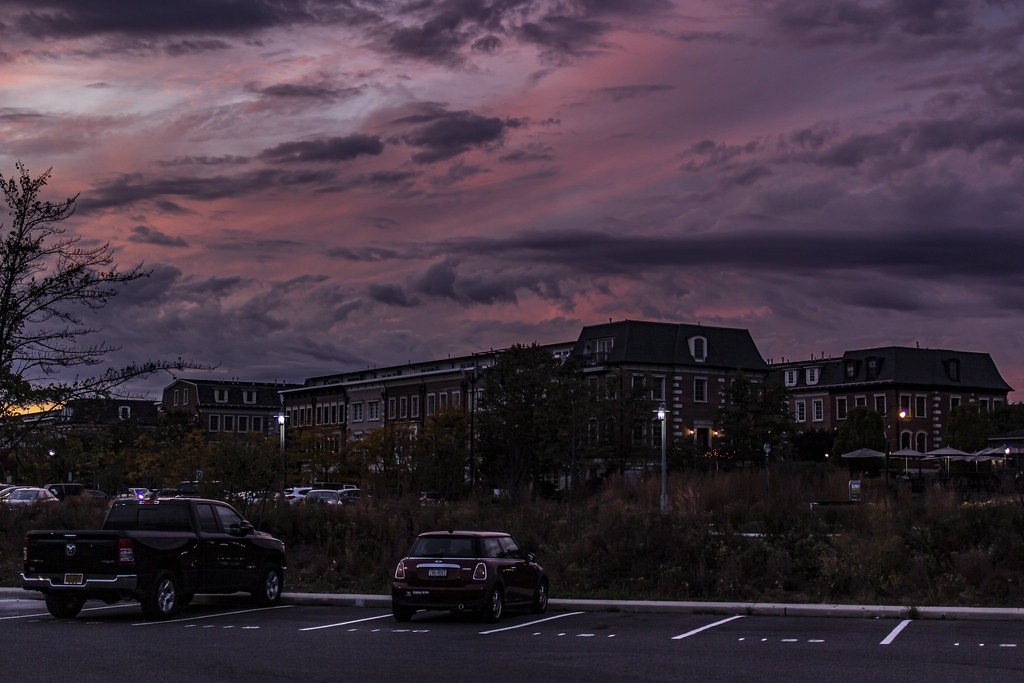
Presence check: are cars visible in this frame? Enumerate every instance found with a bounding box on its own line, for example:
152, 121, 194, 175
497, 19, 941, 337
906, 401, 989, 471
391, 529, 550, 623
117, 479, 375, 505
0, 483, 109, 506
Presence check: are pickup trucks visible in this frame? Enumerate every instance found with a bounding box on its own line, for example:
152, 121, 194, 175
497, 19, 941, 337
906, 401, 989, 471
21, 496, 287, 623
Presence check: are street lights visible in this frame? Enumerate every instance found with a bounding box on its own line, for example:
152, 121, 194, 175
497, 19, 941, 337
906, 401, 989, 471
656, 401, 668, 509
277, 413, 286, 488
